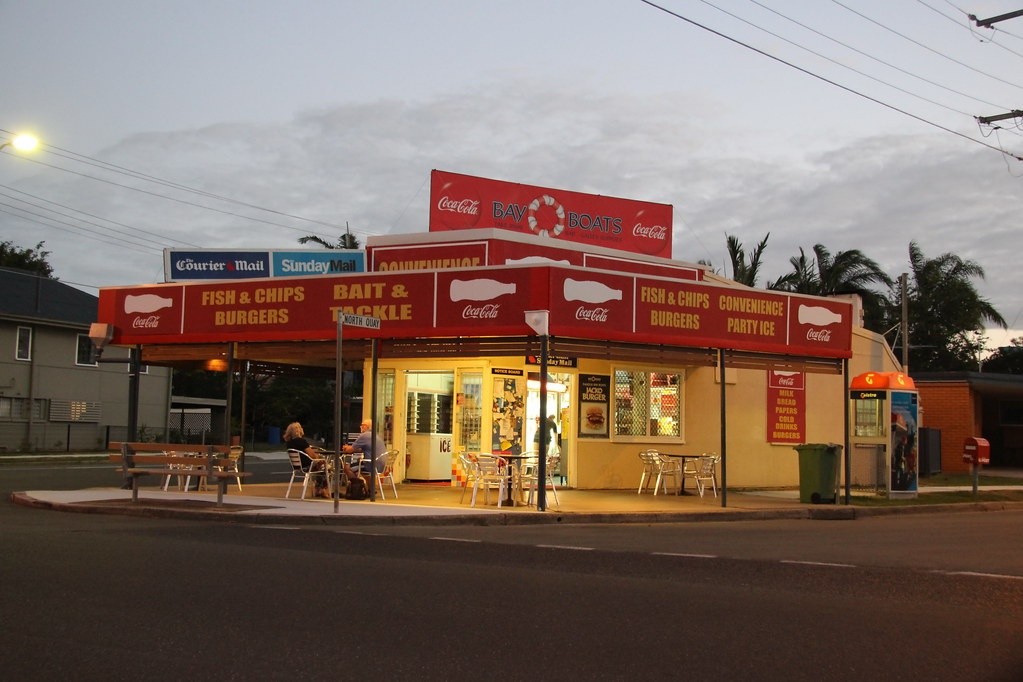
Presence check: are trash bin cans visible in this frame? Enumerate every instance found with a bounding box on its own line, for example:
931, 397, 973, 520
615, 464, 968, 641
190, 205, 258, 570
792, 443, 844, 504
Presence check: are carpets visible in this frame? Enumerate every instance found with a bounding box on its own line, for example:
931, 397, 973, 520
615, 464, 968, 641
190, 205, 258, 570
414, 482, 567, 489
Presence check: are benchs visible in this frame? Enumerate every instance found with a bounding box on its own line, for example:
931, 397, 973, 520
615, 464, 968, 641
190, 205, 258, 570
109, 442, 252, 490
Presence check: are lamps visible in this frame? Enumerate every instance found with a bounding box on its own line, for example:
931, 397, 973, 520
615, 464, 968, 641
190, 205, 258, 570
88, 323, 113, 360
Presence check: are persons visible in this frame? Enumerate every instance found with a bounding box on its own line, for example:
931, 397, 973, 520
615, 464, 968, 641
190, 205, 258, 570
343, 418, 388, 500
533, 415, 559, 464
282, 422, 332, 500
894, 435, 908, 485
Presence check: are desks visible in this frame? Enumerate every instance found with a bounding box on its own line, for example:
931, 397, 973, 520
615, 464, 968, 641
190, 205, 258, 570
184, 451, 218, 491
480, 454, 538, 507
658, 453, 710, 496
316, 450, 364, 499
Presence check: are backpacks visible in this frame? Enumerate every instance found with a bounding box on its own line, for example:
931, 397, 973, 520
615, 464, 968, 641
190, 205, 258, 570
346, 477, 367, 500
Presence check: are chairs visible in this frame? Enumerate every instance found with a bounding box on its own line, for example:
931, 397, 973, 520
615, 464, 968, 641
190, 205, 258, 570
285, 449, 332, 501
357, 449, 399, 501
459, 450, 488, 505
682, 451, 720, 498
470, 453, 516, 508
638, 449, 678, 497
645, 449, 680, 495
162, 450, 207, 492
517, 450, 539, 501
212, 446, 243, 492
518, 444, 561, 508
